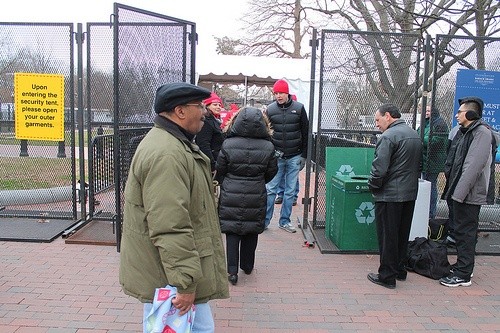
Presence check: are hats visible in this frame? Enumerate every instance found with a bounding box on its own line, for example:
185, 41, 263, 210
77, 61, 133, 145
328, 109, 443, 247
204, 92, 222, 105
273, 80, 289, 94
154, 81, 211, 113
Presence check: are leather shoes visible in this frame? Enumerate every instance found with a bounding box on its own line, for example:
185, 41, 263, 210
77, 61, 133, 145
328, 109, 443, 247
367, 272, 397, 289
228, 274, 238, 284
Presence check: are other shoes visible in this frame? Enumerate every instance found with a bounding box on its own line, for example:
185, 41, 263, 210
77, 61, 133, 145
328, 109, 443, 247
275, 197, 283, 203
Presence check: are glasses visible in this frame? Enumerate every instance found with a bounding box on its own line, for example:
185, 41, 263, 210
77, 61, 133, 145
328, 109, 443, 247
185, 102, 207, 110
458, 109, 467, 113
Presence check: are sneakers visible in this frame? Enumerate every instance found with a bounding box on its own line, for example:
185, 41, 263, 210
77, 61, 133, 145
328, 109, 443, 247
440, 273, 472, 286
279, 223, 296, 232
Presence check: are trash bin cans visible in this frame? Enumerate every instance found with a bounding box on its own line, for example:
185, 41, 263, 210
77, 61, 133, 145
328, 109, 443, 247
329, 175, 379, 250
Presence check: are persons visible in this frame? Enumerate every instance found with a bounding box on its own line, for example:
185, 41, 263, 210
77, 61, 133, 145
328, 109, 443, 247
216, 106, 279, 285
417, 104, 450, 220
119, 82, 230, 333
264, 79, 315, 233
220, 97, 242, 131
366, 103, 424, 289
274, 95, 299, 206
440, 100, 493, 287
196, 91, 227, 194
